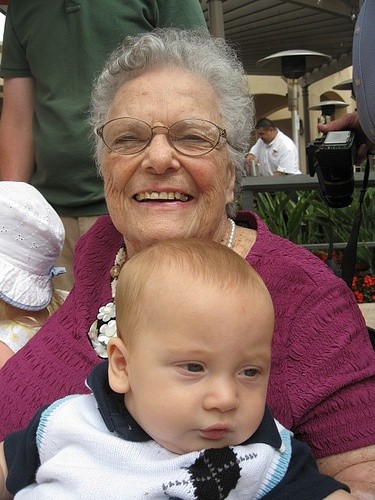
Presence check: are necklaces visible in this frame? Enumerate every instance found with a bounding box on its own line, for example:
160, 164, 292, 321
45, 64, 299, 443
227, 218, 236, 248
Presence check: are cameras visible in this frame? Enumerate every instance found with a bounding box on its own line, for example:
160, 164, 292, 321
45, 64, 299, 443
306, 127, 364, 208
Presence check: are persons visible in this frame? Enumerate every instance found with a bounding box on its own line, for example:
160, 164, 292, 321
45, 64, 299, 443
0, 236, 358, 500
0, 0, 212, 293
244, 118, 302, 204
0, 28, 375, 500
0, 181, 72, 369
316, 0, 375, 166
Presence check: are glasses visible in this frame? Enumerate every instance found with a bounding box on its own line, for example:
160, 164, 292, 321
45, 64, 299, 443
94, 117, 227, 158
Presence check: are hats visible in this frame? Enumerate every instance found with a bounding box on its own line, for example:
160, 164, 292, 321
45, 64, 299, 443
0, 181, 67, 311
